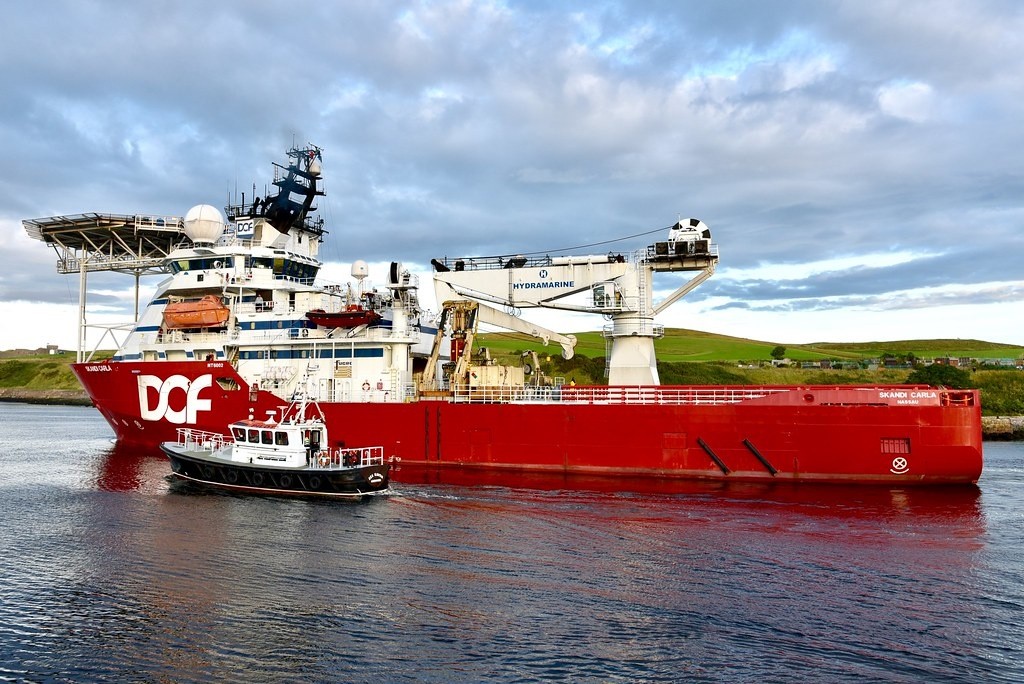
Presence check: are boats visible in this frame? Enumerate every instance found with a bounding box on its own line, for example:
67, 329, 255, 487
160, 294, 233, 327
156, 359, 402, 506
303, 308, 383, 327
23, 132, 989, 486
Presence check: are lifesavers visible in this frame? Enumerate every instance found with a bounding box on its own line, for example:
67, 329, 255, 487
309, 477, 321, 488
252, 473, 263, 485
204, 467, 213, 478
362, 383, 370, 390
318, 453, 330, 465
227, 471, 238, 482
280, 476, 293, 487
